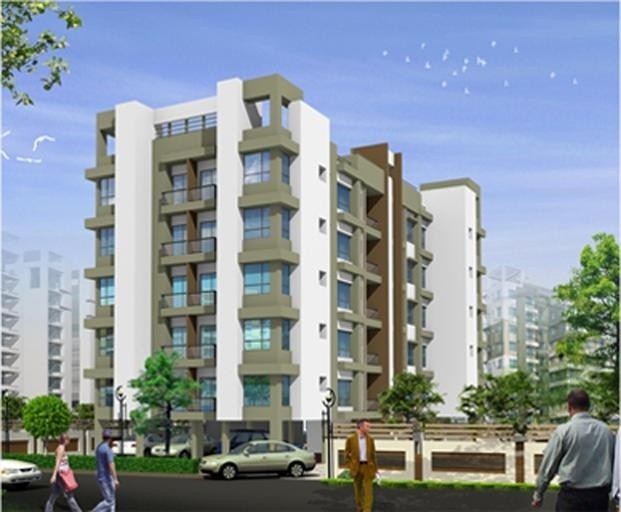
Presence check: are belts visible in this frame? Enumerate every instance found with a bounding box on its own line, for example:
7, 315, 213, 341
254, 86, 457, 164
359, 461, 368, 464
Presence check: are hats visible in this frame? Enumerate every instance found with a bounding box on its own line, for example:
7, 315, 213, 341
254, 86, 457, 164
102, 428, 112, 437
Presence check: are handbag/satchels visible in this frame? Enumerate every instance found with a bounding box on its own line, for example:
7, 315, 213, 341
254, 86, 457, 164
57, 468, 80, 494
